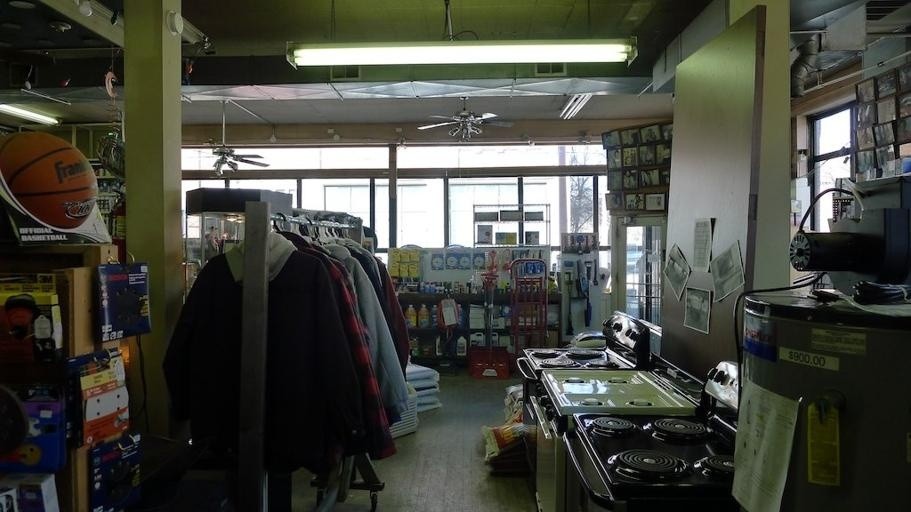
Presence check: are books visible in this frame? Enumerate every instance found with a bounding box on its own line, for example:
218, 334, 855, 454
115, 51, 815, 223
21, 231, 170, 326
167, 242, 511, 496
855, 64, 911, 182
603, 124, 673, 211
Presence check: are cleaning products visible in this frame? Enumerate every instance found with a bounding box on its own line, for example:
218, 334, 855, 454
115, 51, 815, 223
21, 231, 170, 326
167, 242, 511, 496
405, 303, 498, 357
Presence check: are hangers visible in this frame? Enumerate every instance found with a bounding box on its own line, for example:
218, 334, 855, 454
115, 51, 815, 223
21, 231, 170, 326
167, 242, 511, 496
266, 209, 345, 243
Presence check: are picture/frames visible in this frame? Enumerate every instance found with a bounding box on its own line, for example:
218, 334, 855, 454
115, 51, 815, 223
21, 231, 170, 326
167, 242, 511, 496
855, 60, 911, 181
600, 119, 675, 214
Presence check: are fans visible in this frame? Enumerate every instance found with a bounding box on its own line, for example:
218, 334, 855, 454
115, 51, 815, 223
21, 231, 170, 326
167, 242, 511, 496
417, 97, 516, 133
205, 100, 271, 170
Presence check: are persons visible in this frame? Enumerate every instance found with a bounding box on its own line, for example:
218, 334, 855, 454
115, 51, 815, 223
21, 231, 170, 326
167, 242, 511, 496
0, 295, 46, 384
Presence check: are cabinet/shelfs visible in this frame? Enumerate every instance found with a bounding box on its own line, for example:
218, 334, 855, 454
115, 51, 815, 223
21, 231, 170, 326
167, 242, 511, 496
0, 238, 136, 511
395, 289, 561, 380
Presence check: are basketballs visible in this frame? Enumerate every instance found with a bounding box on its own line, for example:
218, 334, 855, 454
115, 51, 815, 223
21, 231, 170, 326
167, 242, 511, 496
0, 129, 99, 230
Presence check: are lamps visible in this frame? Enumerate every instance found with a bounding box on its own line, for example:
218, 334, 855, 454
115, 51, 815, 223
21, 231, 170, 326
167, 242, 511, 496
448, 120, 486, 145
284, 0, 643, 73
210, 154, 241, 177
